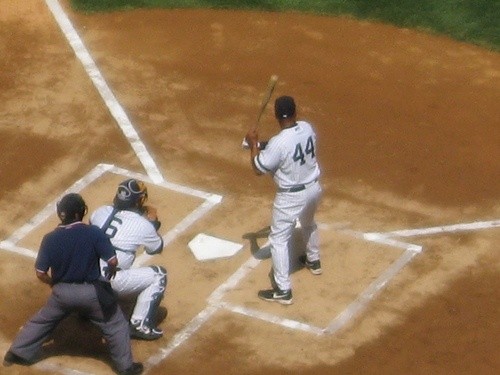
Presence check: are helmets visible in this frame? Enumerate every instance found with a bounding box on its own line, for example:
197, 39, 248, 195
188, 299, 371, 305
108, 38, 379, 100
113, 179, 139, 208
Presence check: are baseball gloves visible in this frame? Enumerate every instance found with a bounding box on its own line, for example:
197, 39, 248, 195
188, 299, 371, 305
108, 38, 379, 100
142, 205, 158, 223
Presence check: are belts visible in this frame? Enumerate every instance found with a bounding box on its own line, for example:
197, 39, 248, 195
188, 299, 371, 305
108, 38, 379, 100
60, 279, 92, 285
280, 179, 317, 192
103, 266, 121, 271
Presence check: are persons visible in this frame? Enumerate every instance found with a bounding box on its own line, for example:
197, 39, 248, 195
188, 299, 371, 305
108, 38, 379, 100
246, 96, 322, 305
90, 179, 167, 341
2, 193, 144, 375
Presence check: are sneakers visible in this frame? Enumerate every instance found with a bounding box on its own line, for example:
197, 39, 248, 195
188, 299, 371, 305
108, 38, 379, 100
298, 256, 322, 275
119, 362, 142, 375
2, 352, 17, 364
259, 287, 293, 304
128, 322, 163, 339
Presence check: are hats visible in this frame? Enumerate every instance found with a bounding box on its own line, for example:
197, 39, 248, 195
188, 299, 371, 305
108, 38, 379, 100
59, 193, 84, 212
275, 96, 294, 117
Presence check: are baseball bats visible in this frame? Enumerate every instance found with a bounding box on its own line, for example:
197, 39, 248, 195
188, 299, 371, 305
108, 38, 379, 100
253, 75, 278, 131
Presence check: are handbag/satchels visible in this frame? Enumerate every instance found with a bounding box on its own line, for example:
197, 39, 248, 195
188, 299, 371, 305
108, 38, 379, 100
95, 280, 117, 321
